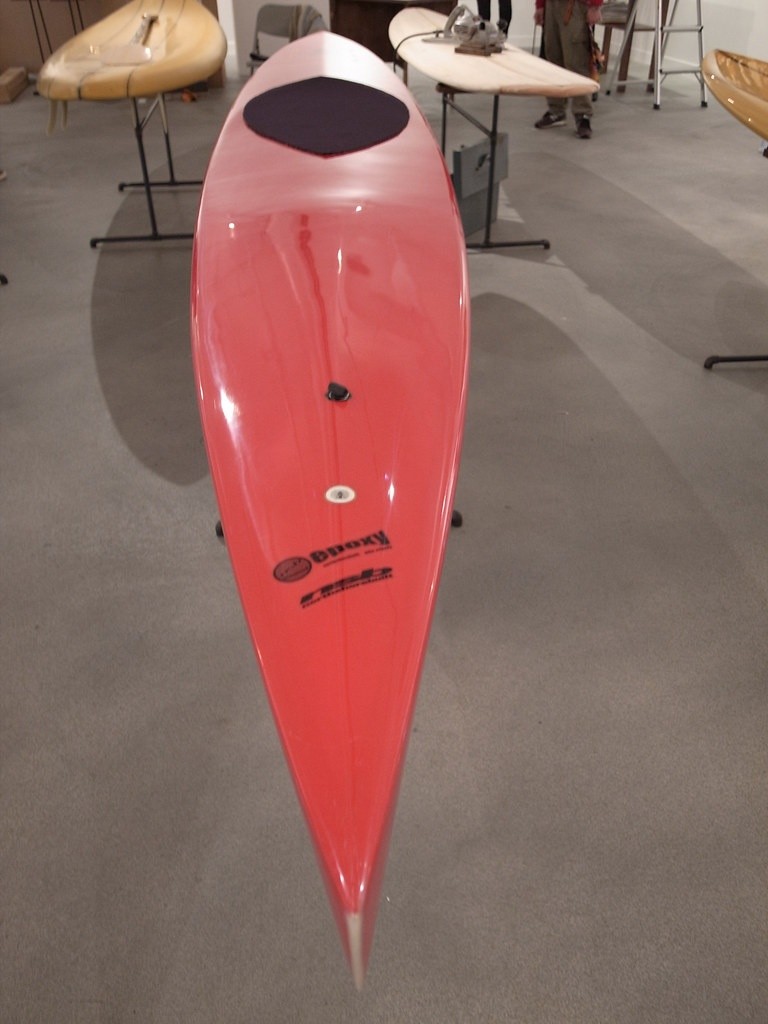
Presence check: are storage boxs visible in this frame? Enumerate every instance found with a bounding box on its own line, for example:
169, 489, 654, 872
449, 130, 508, 238
0, 65, 28, 105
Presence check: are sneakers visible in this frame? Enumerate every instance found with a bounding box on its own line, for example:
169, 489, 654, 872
534, 112, 568, 129
574, 113, 592, 138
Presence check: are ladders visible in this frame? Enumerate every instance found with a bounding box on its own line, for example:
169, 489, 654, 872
605, 0, 710, 109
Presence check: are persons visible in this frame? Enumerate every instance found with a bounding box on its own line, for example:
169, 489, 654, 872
477, 0, 512, 42
533, 0, 604, 140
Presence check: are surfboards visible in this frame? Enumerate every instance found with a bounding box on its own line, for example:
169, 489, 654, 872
179, 30, 473, 994
36, 0, 225, 101
700, 49, 767, 134
388, 8, 602, 99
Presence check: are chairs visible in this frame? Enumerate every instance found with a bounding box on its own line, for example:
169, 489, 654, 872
246, 3, 328, 78
597, 0, 668, 94
